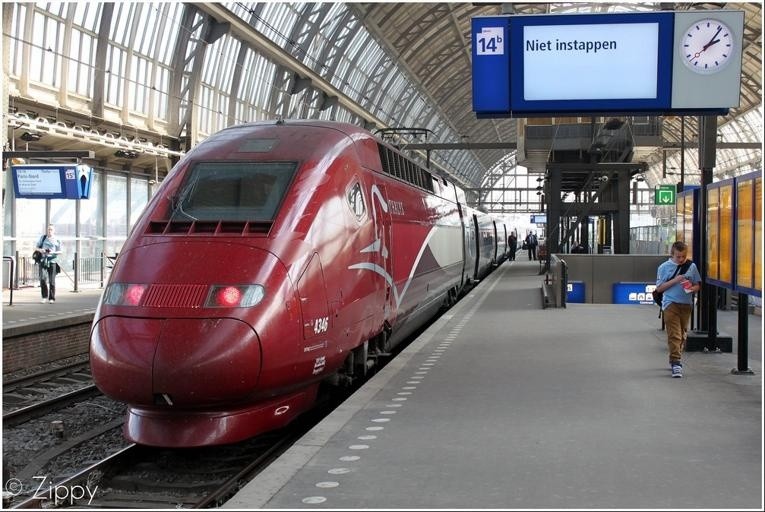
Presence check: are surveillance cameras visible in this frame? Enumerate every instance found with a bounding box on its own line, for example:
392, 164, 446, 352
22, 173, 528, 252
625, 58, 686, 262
602, 175, 609, 182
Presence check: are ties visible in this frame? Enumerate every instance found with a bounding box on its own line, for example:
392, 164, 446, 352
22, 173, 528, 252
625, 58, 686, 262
531, 236, 532, 243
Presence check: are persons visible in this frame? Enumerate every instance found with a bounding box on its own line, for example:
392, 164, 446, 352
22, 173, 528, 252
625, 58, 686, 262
656, 241, 703, 378
521, 230, 538, 261
508, 231, 517, 261
34, 223, 64, 305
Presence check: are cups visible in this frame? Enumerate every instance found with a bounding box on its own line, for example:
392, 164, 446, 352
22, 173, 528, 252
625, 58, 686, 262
680, 279, 694, 294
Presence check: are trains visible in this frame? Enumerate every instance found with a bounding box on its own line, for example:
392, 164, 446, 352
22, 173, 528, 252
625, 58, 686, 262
89, 117, 536, 448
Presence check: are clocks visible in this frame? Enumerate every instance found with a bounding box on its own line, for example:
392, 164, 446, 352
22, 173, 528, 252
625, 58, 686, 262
677, 17, 734, 78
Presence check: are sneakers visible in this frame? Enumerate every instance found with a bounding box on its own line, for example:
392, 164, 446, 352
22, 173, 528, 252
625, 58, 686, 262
41, 298, 54, 304
673, 363, 682, 378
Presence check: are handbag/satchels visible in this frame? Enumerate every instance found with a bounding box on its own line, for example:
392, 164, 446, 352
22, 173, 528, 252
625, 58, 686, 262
33, 251, 40, 259
522, 244, 529, 250
653, 290, 662, 305
